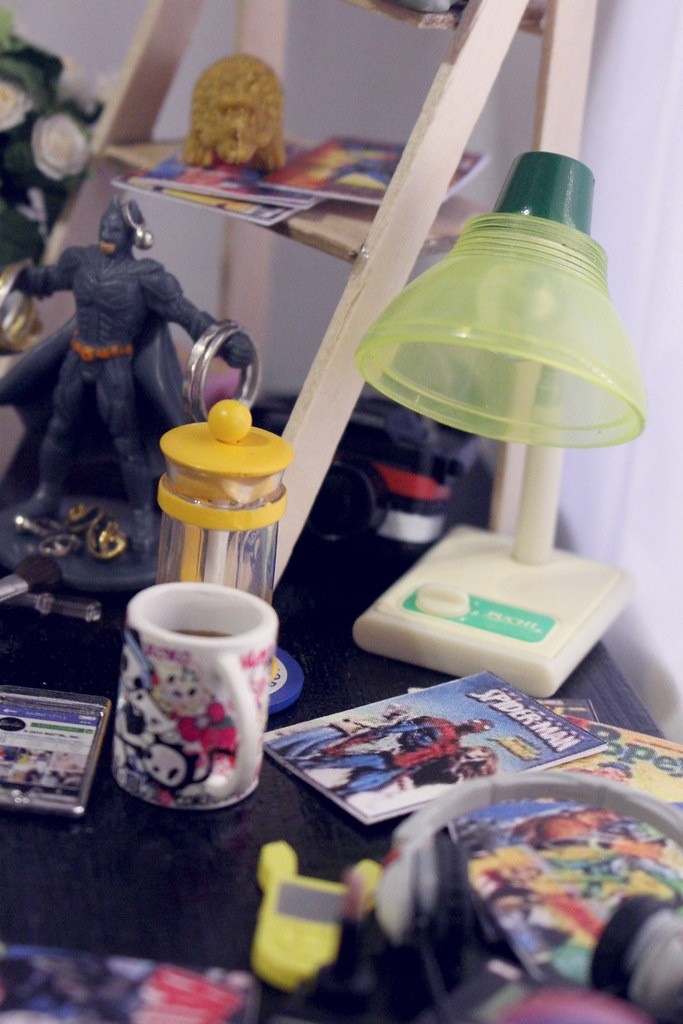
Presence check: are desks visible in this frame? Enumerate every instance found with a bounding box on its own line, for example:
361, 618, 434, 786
0, 390, 683, 1021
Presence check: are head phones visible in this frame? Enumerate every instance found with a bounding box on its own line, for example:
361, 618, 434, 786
371, 769, 683, 1024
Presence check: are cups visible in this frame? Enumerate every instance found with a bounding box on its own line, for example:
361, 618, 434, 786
110, 582, 278, 809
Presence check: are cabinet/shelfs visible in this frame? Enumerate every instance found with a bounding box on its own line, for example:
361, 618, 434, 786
27, 3, 604, 623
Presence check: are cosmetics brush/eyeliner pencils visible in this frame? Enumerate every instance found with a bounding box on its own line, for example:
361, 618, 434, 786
0, 555, 59, 607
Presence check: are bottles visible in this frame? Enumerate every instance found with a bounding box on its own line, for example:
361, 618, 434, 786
154, 397, 296, 607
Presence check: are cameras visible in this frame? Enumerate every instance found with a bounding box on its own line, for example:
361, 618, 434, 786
249, 392, 480, 558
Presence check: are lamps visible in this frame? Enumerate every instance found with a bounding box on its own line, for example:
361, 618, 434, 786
351, 153, 648, 696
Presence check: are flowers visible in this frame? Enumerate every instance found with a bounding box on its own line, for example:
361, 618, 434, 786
0, 9, 105, 274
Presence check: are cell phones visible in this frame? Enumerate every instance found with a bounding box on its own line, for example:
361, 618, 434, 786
1, 683, 112, 818
